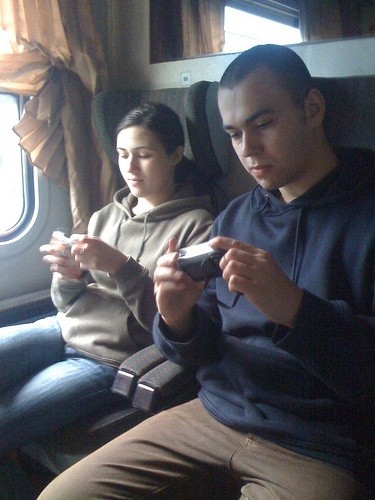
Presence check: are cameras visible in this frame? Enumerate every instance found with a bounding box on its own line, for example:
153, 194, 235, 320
51, 239, 76, 250
177, 242, 226, 282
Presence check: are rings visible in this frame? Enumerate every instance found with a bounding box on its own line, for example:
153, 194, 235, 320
81, 248, 87, 255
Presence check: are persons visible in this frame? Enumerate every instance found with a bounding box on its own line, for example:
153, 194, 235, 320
0, 101, 215, 459
36, 44, 375, 500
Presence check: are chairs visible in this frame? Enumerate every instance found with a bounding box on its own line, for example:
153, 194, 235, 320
16, 74, 374, 499
